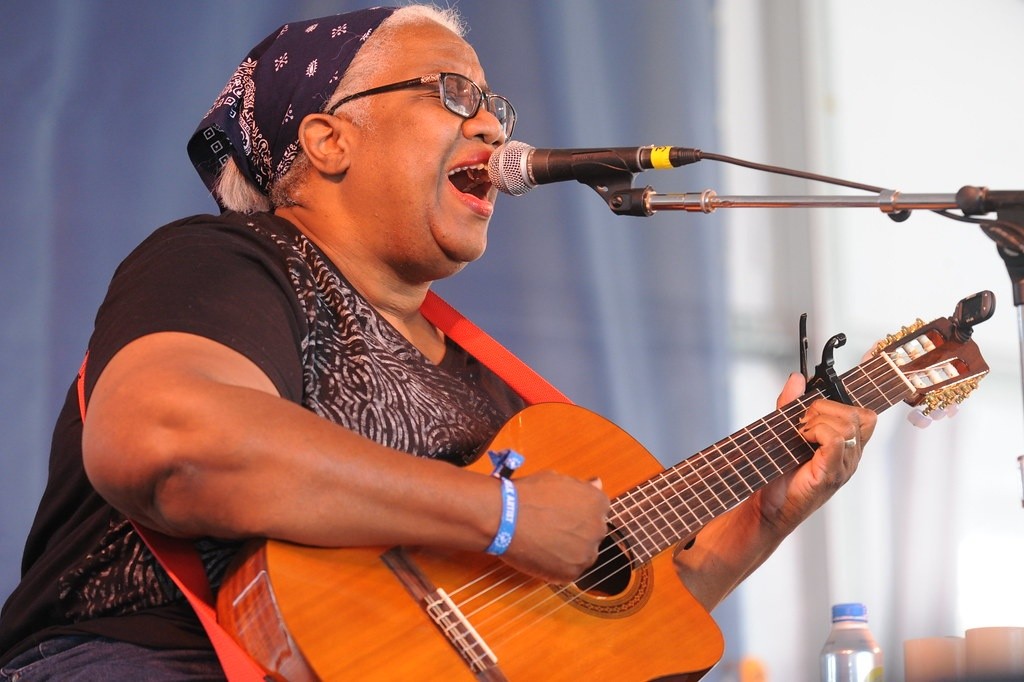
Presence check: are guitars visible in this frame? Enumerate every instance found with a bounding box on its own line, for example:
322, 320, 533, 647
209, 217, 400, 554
217, 288, 995, 680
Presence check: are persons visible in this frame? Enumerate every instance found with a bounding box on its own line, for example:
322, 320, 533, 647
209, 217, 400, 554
0, 5, 877, 682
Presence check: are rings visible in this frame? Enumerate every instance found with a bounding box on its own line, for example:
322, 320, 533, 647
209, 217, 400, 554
845, 437, 857, 448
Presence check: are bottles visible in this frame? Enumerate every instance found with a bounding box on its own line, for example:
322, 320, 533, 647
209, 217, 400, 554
819, 603, 884, 682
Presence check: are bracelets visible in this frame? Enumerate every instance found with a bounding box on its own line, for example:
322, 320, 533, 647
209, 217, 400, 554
484, 448, 526, 556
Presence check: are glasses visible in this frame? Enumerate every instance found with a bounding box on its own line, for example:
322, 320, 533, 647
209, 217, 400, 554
325, 71, 518, 143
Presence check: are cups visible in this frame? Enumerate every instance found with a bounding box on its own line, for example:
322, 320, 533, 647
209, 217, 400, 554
903, 634, 962, 681
964, 626, 1023, 681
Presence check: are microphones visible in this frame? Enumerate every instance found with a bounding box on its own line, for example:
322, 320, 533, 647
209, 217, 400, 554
488, 141, 702, 196
